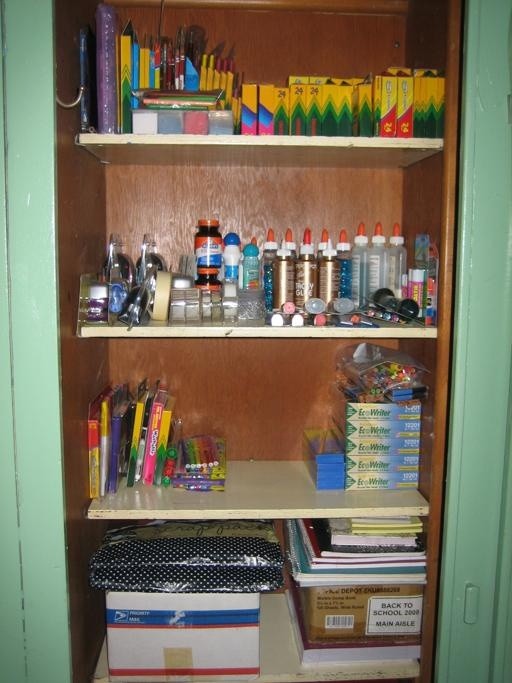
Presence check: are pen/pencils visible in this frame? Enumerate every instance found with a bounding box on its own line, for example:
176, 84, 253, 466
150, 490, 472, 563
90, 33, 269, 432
173, 436, 226, 493
336, 359, 418, 403
141, 89, 220, 111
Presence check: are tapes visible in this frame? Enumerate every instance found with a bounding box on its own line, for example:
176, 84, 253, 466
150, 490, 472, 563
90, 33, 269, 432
151, 271, 172, 322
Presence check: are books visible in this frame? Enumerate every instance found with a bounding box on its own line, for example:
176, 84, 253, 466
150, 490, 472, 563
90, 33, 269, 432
289, 515, 428, 588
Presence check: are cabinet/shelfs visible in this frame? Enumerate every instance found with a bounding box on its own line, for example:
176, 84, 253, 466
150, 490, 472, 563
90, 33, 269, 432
0, 0, 511, 683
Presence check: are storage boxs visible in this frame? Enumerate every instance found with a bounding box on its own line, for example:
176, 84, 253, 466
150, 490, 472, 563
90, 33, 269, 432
104, 587, 261, 679
283, 521, 425, 650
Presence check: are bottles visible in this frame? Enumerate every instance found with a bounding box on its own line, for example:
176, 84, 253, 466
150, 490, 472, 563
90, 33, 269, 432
193, 218, 408, 315
